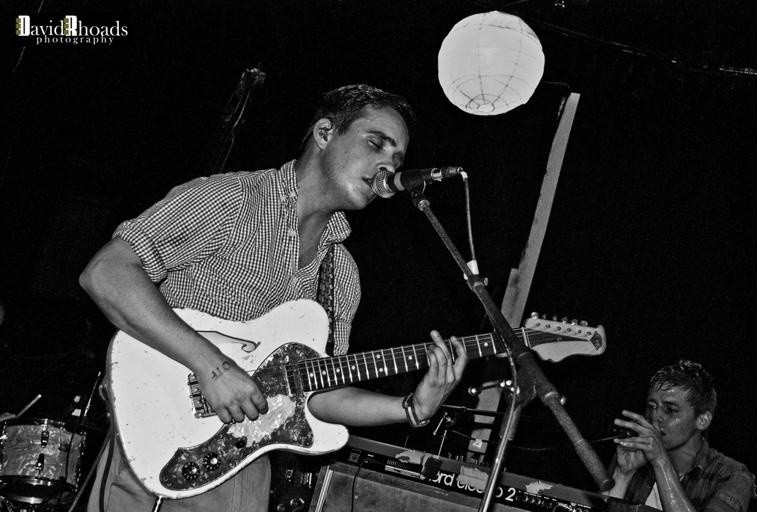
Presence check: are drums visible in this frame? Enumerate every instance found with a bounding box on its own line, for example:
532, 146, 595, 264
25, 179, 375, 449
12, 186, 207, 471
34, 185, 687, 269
0, 414, 88, 509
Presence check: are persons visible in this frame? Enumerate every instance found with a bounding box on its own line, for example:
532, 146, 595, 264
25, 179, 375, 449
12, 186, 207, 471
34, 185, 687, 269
602, 359, 753, 512
77, 81, 470, 512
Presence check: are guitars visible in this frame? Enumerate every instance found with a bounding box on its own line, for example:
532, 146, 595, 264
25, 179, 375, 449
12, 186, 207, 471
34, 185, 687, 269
103, 300, 607, 501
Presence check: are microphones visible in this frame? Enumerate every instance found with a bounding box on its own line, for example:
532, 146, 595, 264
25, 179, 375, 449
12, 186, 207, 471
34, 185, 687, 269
237, 57, 273, 88
371, 163, 466, 201
612, 423, 661, 442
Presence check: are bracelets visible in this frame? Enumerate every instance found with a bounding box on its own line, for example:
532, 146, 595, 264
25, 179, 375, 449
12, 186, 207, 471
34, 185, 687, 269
404, 392, 431, 428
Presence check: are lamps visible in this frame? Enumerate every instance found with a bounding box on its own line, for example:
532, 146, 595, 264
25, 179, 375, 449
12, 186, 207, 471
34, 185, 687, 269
436, 12, 547, 118
